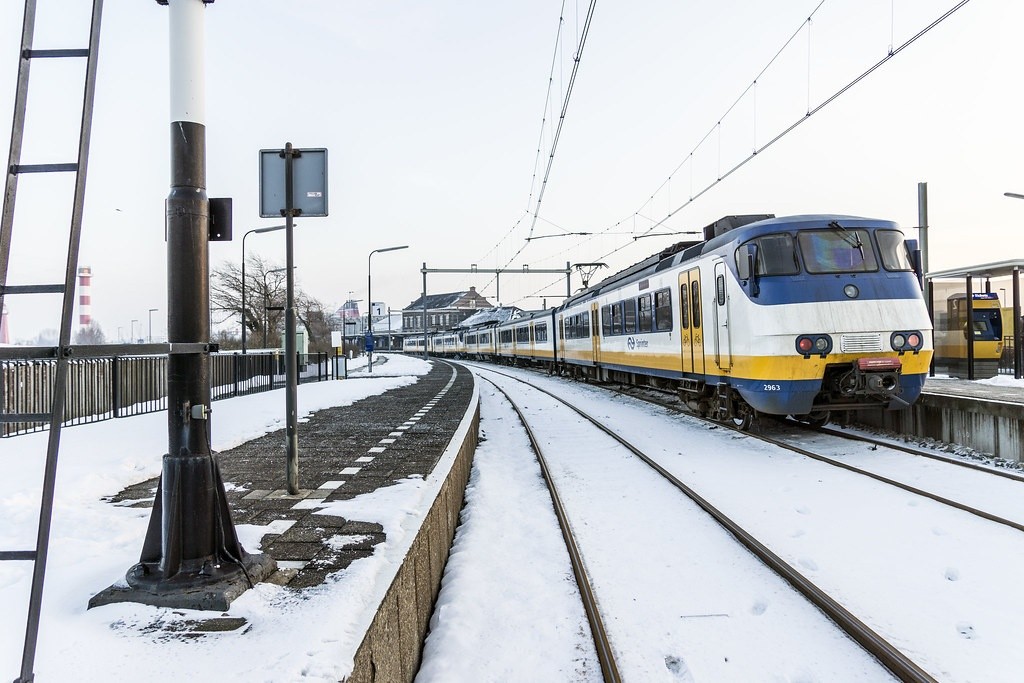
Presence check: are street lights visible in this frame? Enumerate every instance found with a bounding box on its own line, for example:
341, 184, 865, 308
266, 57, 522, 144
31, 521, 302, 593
131, 320, 138, 343
263, 267, 297, 348
149, 309, 159, 342
368, 246, 409, 372
343, 300, 362, 355
118, 327, 122, 341
242, 223, 297, 354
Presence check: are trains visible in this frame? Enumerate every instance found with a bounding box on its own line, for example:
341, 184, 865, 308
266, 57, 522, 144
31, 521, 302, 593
404, 213, 936, 430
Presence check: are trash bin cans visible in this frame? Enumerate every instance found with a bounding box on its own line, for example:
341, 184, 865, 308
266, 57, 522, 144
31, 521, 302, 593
332, 354, 347, 377
